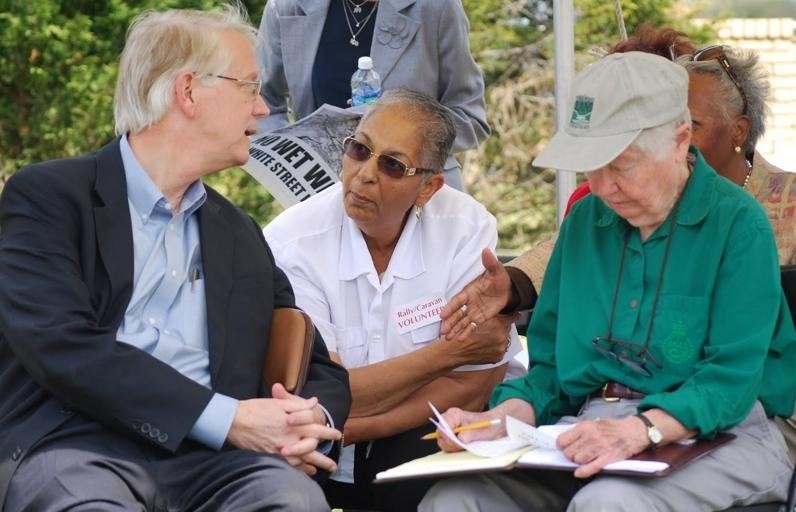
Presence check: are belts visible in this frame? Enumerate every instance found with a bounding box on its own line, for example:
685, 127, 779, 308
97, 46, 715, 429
589, 382, 645, 398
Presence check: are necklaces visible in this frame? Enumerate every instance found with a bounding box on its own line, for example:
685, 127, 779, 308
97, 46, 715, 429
740, 159, 754, 189
338, 1, 381, 48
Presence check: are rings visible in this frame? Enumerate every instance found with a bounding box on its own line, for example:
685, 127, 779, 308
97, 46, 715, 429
460, 304, 469, 315
470, 321, 478, 327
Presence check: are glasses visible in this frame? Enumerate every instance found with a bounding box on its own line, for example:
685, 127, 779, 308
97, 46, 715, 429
691, 46, 747, 114
207, 72, 261, 94
342, 135, 433, 178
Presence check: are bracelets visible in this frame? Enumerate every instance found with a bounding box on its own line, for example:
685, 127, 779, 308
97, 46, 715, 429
318, 422, 330, 444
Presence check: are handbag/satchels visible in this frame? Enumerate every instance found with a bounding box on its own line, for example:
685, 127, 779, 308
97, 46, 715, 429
261, 309, 314, 395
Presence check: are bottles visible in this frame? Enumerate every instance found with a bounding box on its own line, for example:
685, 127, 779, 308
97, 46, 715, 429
351, 56, 382, 107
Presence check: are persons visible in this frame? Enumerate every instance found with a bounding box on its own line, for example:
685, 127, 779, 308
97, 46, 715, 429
411, 49, 796, 512
438, 42, 796, 342
0, 1, 355, 511
248, 1, 495, 208
257, 86, 527, 511
556, 17, 705, 240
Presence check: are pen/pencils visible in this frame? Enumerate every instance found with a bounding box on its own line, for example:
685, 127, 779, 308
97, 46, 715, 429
420, 419, 501, 440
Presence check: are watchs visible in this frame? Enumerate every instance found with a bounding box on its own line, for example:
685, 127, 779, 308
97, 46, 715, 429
635, 412, 665, 452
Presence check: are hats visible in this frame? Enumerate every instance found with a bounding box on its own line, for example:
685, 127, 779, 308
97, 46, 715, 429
532, 51, 689, 173
